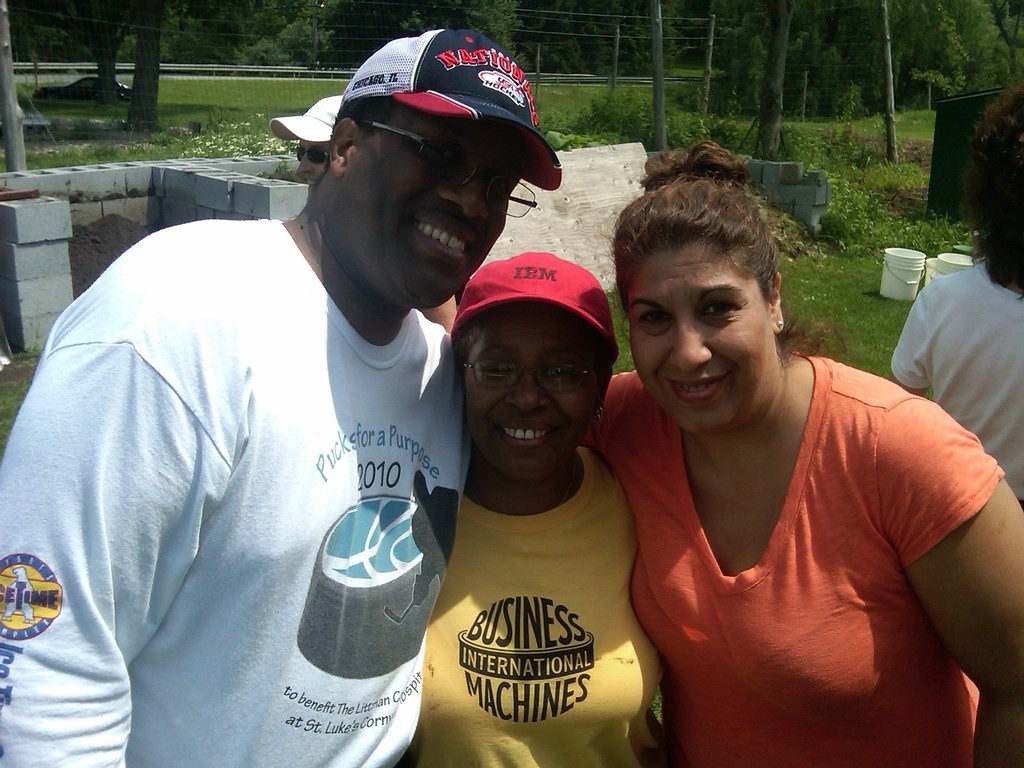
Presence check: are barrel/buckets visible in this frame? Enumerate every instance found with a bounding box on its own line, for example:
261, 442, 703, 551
880, 245, 984, 301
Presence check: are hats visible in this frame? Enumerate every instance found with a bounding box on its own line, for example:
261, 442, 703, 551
451, 252, 619, 365
337, 28, 563, 190
269, 95, 345, 142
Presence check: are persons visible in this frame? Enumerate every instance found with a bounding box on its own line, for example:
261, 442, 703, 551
1, 28, 1024, 768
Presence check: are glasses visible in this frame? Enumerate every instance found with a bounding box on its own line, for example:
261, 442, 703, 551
462, 357, 606, 393
352, 117, 538, 218
295, 145, 332, 164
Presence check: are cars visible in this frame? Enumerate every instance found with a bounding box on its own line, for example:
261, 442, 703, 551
33, 77, 132, 102
